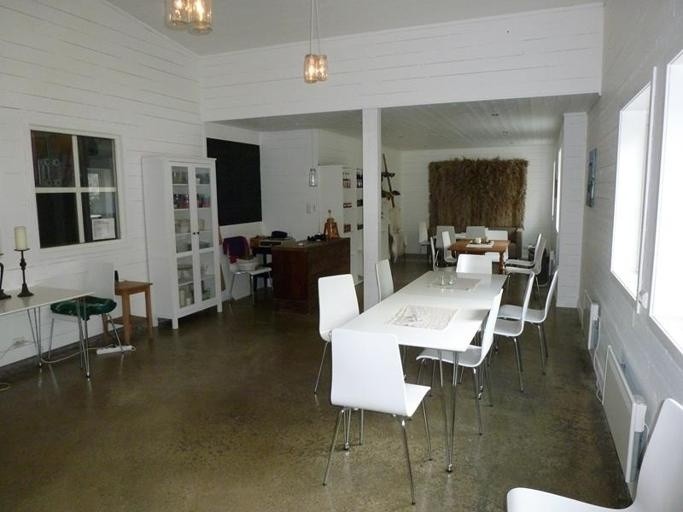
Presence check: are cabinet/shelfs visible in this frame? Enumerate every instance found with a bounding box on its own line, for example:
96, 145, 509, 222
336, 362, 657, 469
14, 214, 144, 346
318, 163, 363, 289
137, 150, 225, 331
271, 234, 351, 314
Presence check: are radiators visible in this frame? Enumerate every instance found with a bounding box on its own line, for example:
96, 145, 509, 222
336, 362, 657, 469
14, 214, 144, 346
574, 286, 600, 355
596, 344, 645, 491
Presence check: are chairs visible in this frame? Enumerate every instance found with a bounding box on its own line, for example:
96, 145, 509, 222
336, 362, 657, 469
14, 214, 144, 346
307, 271, 405, 404
504, 394, 682, 512
382, 219, 564, 400
412, 286, 504, 446
246, 231, 276, 301
224, 230, 270, 306
319, 324, 438, 506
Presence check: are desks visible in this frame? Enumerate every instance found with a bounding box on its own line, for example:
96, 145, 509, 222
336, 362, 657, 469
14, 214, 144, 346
321, 268, 512, 473
0, 284, 98, 386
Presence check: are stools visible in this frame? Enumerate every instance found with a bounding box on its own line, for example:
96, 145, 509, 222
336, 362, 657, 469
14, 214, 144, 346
43, 294, 118, 379
101, 278, 154, 349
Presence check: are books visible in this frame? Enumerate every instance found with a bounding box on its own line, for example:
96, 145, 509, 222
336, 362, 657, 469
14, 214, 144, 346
174, 192, 210, 209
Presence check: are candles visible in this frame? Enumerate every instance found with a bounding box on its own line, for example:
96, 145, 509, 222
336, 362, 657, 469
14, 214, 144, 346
13, 225, 26, 249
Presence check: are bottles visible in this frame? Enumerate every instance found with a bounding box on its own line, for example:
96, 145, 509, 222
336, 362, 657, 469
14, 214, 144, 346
309, 168, 317, 187
439, 272, 456, 286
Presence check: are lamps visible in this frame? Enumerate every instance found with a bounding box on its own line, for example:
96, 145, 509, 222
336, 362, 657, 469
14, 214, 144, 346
303, 1, 327, 82
162, 0, 215, 35
